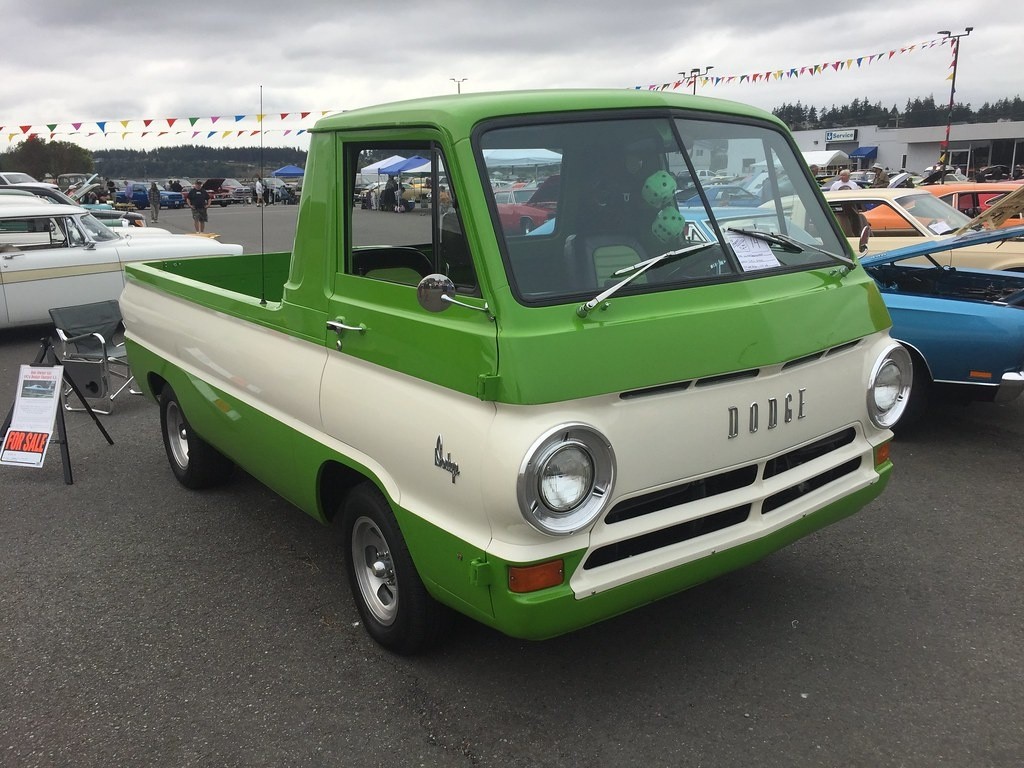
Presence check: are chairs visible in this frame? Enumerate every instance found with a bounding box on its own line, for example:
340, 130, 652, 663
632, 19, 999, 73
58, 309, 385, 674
352, 247, 434, 287
49, 299, 143, 415
964, 206, 981, 218
847, 203, 874, 237
564, 230, 652, 290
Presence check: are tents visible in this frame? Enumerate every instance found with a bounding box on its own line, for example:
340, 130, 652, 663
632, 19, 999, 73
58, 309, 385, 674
378, 155, 431, 212
482, 148, 564, 188
361, 155, 408, 209
400, 155, 445, 209
753, 150, 850, 174
271, 165, 305, 203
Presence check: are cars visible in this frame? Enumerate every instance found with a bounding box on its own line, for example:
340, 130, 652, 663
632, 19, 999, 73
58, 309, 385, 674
0, 172, 302, 227
863, 182, 1023, 230
673, 165, 1024, 244
354, 168, 561, 236
719, 189, 1024, 274
858, 225, 1024, 439
0, 204, 243, 334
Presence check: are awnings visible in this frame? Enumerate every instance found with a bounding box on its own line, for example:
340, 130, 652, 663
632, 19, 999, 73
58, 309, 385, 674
849, 147, 878, 159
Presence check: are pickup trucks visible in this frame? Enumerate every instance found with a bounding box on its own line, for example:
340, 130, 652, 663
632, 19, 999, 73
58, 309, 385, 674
120, 86, 915, 655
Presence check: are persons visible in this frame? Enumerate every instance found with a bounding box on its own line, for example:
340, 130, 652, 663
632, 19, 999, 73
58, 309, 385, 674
931, 164, 962, 175
439, 186, 451, 213
394, 187, 410, 211
187, 179, 211, 234
870, 163, 890, 188
278, 185, 294, 205
68, 185, 79, 204
370, 189, 376, 209
105, 176, 116, 207
809, 165, 819, 177
256, 177, 267, 207
124, 180, 133, 203
167, 179, 182, 192
148, 182, 160, 220
830, 169, 862, 190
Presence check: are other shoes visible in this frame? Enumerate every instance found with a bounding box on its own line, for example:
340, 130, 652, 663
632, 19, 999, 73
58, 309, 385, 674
265, 203, 269, 206
154, 219, 159, 223
257, 205, 261, 207
151, 219, 155, 223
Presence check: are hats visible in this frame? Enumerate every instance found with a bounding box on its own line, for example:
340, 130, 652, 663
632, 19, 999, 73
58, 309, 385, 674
195, 179, 203, 184
872, 162, 883, 170
